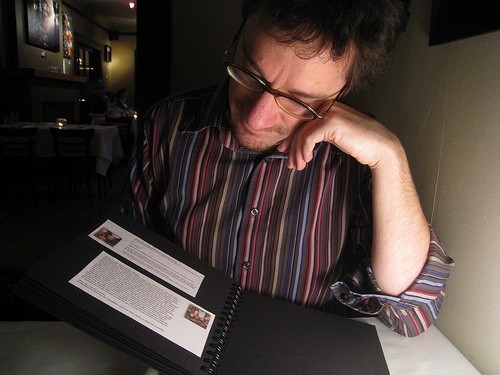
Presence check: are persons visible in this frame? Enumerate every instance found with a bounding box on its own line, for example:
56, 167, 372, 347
120, 0, 456, 337
34, 0, 60, 45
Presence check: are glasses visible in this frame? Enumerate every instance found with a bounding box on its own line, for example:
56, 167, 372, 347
223, 16, 352, 121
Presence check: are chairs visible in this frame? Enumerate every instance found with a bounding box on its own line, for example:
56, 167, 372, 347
0, 116, 135, 209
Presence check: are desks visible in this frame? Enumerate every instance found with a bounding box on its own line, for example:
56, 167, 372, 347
0, 122, 124, 204
0, 316, 485, 375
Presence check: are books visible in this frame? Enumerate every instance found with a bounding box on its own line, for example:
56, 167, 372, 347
18, 209, 390, 375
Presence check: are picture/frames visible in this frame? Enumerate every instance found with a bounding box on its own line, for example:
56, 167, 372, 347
23, 0, 60, 52
105, 45, 111, 62
